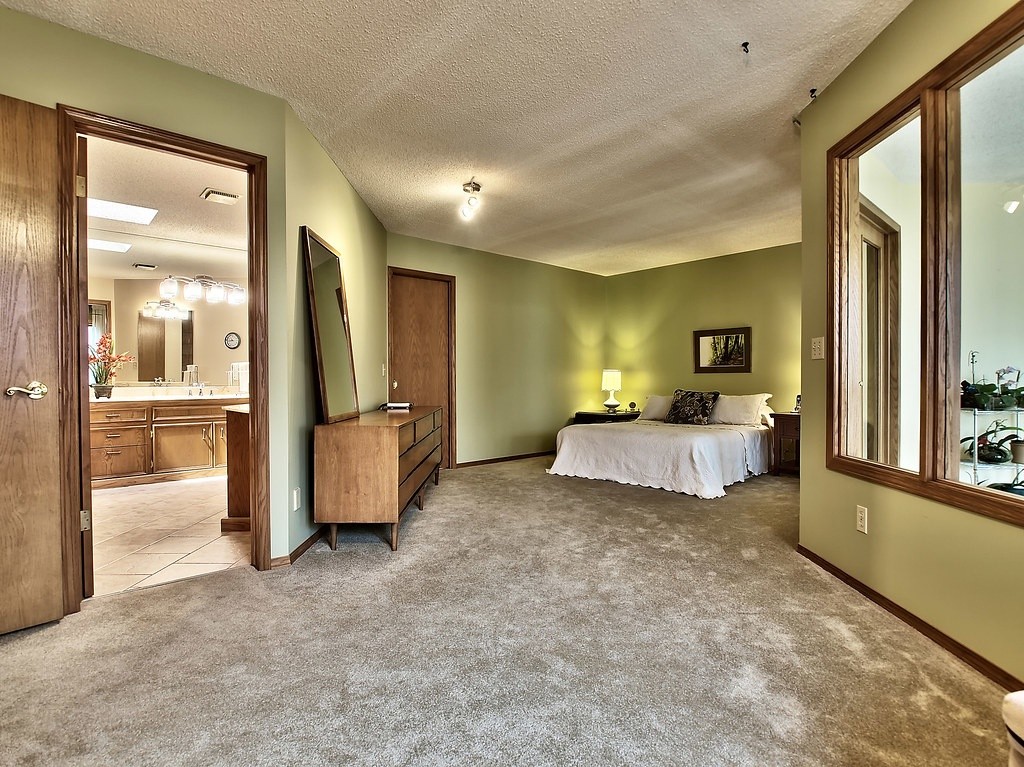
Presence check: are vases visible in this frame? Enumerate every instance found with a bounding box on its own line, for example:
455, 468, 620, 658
90, 383, 113, 399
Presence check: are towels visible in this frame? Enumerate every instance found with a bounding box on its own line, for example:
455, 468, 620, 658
183, 371, 190, 382
239, 362, 248, 391
186, 364, 197, 381
227, 364, 238, 387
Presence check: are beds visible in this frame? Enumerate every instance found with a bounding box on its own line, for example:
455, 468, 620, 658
546, 420, 773, 499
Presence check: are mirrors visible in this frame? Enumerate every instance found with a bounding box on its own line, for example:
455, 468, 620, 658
304, 225, 361, 423
114, 278, 249, 387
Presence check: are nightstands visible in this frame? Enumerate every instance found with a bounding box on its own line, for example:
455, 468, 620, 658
769, 410, 800, 475
574, 412, 639, 424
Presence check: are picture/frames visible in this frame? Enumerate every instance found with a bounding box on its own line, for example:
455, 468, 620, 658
693, 327, 753, 374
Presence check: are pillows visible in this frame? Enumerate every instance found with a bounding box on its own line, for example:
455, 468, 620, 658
638, 395, 672, 419
666, 389, 720, 424
710, 393, 772, 426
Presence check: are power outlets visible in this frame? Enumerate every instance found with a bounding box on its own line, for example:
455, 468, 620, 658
856, 505, 867, 535
293, 488, 302, 511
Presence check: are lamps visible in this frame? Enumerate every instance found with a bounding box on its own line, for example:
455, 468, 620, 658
159, 275, 245, 305
461, 180, 482, 218
143, 300, 189, 321
602, 369, 621, 413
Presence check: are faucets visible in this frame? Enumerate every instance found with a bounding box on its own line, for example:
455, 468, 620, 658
199, 387, 203, 396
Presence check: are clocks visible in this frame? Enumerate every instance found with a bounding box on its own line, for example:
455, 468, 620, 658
225, 332, 241, 349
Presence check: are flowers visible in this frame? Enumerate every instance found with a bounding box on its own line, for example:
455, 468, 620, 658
88, 333, 136, 384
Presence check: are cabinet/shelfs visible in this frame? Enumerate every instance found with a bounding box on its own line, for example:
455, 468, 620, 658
151, 401, 226, 472
313, 405, 444, 551
960, 408, 1024, 488
90, 402, 152, 488
